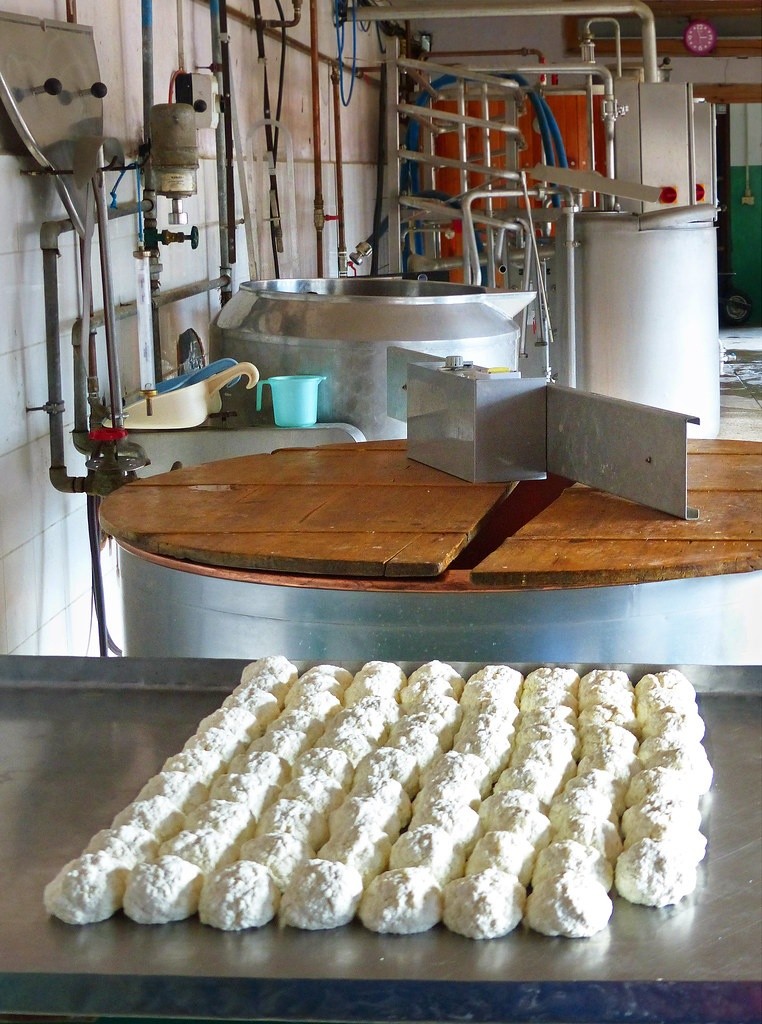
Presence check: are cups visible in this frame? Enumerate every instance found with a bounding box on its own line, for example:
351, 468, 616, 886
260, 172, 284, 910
256, 372, 326, 427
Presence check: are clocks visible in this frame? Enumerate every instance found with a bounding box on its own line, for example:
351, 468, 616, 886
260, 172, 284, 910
683, 21, 717, 55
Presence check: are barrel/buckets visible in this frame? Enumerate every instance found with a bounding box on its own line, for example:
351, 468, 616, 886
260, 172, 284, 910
214, 276, 537, 437
113, 438, 762, 675
